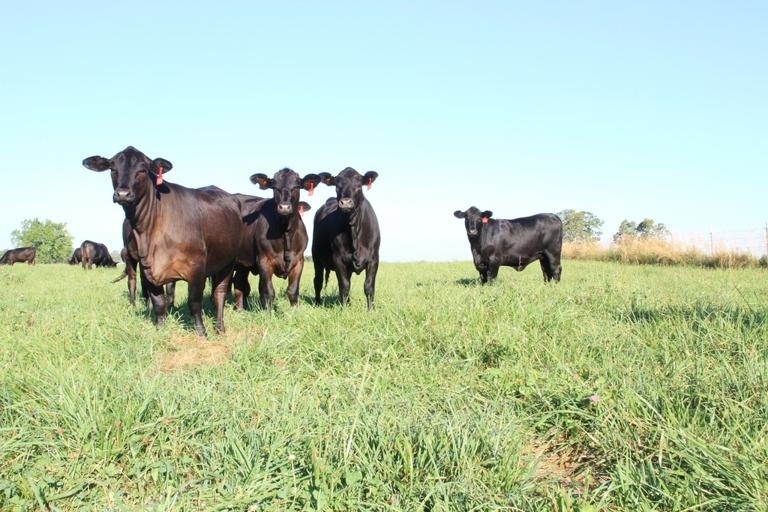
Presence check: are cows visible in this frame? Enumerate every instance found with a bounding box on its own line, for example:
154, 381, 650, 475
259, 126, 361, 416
0, 247, 36, 266
233, 169, 321, 314
68, 248, 82, 265
453, 206, 563, 286
81, 146, 242, 339
311, 167, 380, 311
81, 240, 120, 270
